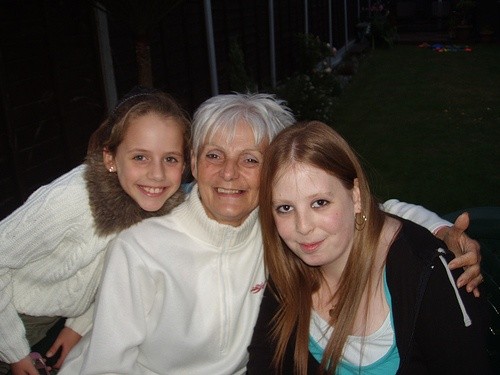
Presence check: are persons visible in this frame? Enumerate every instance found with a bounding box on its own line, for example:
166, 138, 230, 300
84, 94, 483, 375
247, 119, 493, 375
0, 96, 187, 375
